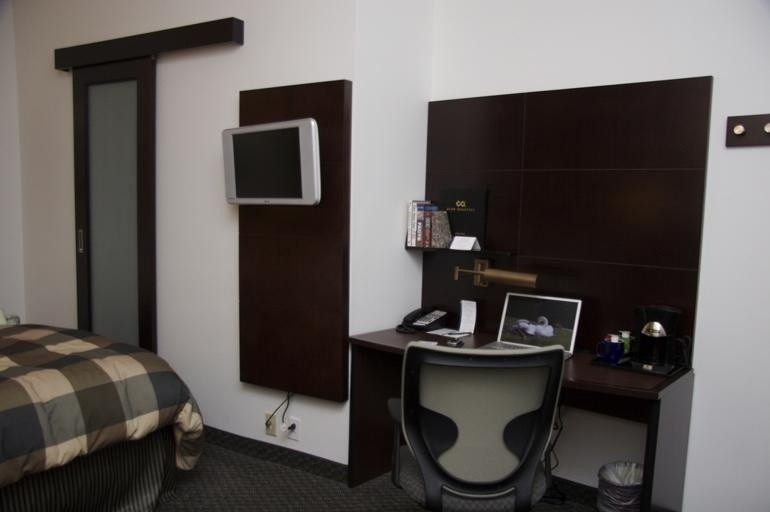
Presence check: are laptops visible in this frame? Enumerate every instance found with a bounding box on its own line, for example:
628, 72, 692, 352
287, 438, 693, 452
476, 292, 582, 362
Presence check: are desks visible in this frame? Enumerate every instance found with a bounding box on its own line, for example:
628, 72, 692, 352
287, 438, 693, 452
347, 321, 696, 512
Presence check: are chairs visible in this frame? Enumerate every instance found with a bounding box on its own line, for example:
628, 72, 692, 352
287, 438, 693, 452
388, 340, 564, 511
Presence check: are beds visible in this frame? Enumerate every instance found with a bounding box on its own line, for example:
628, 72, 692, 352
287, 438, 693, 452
0, 317, 206, 511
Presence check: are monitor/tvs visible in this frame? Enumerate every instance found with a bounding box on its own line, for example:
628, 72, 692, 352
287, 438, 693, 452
222, 117, 321, 205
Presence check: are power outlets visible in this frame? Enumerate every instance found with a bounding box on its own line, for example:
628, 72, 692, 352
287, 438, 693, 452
264, 411, 278, 439
287, 416, 303, 442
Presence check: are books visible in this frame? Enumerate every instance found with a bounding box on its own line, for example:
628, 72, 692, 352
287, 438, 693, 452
406, 199, 452, 248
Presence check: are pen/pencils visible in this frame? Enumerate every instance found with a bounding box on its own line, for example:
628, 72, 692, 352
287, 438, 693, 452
448, 332, 472, 335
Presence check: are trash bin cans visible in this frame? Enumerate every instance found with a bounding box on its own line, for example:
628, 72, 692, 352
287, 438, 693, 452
597, 461, 644, 512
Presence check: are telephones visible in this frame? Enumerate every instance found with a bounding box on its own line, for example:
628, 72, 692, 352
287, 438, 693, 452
403, 306, 448, 332
